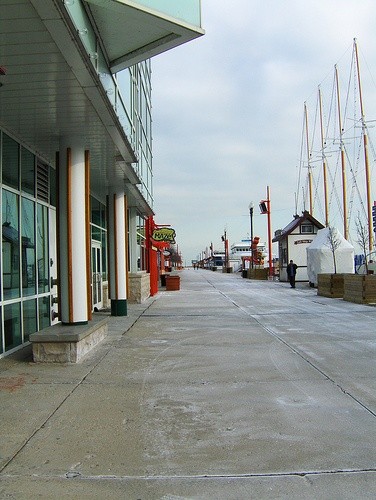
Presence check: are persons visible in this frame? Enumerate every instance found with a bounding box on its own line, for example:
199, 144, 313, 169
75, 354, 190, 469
196, 265, 198, 270
287, 259, 298, 288
194, 263, 195, 270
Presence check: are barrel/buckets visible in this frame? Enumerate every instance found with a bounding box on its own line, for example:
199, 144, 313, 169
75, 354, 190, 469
268, 276, 273, 281
111, 299, 127, 316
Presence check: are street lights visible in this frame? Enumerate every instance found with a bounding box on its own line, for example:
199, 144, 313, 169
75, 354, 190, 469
248, 201, 254, 269
